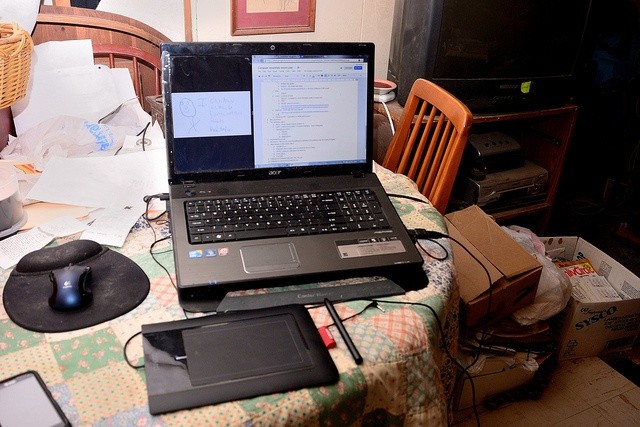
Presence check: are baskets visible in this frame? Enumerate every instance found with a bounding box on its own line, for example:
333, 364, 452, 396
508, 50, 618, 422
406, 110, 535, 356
0, 22, 34, 110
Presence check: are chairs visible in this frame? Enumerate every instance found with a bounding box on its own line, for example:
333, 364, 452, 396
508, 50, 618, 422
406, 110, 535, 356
92, 43, 165, 116
382, 77, 473, 216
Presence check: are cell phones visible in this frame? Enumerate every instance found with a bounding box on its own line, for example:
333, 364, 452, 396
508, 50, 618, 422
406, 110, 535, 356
0, 370, 72, 427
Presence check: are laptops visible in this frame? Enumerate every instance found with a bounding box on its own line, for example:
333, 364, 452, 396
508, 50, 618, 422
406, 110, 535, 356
160, 42, 424, 288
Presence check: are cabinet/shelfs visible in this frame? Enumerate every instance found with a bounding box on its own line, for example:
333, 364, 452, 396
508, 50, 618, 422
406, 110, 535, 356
372, 102, 579, 236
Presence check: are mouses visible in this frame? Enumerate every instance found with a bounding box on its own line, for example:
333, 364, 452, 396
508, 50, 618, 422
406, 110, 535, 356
48, 263, 94, 314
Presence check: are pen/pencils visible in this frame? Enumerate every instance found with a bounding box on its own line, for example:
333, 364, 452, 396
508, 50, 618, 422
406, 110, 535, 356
325, 300, 363, 365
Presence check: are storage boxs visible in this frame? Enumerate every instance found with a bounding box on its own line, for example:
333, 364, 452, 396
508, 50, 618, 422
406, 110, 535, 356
544, 237, 640, 358
445, 205, 544, 325
457, 357, 639, 426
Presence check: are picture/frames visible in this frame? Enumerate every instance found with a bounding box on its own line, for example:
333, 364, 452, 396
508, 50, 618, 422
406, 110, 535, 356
230, 0, 316, 36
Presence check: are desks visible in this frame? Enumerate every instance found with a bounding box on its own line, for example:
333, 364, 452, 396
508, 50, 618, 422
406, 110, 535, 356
2, 161, 453, 427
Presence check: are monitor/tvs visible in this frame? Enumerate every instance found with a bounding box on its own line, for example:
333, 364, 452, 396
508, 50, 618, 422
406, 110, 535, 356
387, 0, 602, 113
